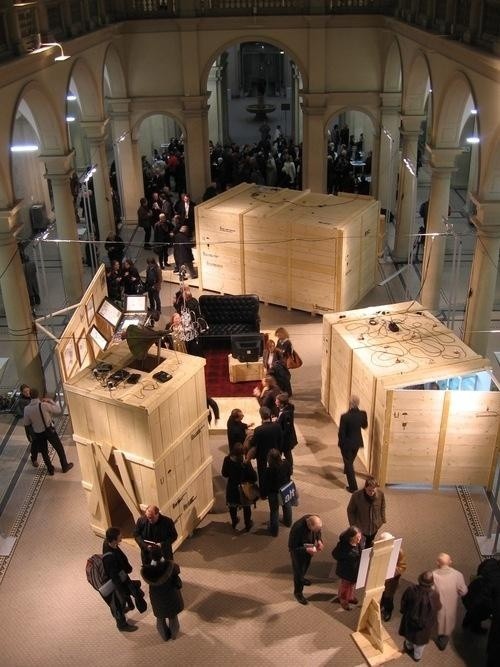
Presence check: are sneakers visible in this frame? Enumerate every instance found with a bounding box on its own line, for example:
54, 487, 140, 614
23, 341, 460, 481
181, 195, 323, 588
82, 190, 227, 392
404, 640, 421, 662
383, 604, 394, 621
143, 242, 171, 270
117, 621, 140, 633
346, 486, 355, 492
340, 596, 359, 610
32, 456, 75, 475
227, 513, 294, 536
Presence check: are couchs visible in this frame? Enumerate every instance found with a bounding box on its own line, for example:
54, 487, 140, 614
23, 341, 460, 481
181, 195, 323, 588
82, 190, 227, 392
199, 294, 261, 350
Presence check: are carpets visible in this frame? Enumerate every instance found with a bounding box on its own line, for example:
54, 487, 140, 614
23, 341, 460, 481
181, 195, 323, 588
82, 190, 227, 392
204, 349, 263, 397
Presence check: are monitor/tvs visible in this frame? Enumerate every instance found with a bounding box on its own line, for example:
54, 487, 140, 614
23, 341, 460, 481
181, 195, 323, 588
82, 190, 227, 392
230, 333, 265, 362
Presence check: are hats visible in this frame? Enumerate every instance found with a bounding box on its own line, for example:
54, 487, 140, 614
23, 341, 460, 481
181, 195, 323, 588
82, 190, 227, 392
418, 569, 436, 588
231, 443, 248, 457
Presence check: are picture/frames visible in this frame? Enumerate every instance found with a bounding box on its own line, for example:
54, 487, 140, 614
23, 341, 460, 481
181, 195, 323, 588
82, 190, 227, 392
61, 333, 78, 379
77, 328, 89, 367
86, 294, 96, 326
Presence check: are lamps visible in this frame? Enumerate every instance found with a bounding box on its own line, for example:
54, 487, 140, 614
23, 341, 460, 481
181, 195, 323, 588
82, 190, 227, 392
114, 130, 132, 143
78, 163, 98, 184
39, 41, 71, 62
31, 219, 56, 243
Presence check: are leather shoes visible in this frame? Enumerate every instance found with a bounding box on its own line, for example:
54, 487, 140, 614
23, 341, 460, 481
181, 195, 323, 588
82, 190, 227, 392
294, 578, 312, 605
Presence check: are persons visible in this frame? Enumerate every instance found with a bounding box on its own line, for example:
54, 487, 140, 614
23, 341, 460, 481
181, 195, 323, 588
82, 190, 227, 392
15, 125, 499, 660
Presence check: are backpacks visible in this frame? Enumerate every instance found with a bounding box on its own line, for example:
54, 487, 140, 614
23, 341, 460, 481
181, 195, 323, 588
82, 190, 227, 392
85, 553, 113, 590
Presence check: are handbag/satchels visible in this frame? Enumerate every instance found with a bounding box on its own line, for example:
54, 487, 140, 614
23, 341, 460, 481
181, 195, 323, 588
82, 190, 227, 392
43, 424, 58, 439
284, 340, 302, 373
279, 480, 298, 507
239, 481, 261, 506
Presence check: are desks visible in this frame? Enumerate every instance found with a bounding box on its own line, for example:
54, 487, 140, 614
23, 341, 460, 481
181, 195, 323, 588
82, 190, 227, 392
228, 355, 265, 384
